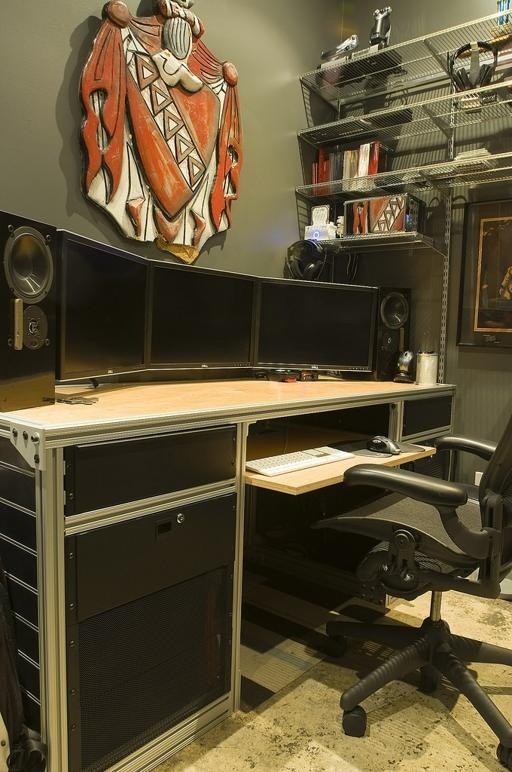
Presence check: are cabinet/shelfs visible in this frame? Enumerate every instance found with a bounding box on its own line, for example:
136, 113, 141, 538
294, 8, 512, 258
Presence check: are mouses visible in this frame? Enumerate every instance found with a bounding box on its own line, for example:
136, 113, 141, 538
367, 434, 403, 454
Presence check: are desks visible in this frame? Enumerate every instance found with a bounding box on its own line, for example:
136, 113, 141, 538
0, 374, 458, 772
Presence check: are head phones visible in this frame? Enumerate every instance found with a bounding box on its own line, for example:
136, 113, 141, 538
281, 238, 331, 281
448, 41, 499, 93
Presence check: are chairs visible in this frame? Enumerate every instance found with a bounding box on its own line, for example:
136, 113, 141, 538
309, 415, 512, 770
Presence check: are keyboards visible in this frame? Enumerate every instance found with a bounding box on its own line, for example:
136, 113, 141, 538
245, 446, 355, 479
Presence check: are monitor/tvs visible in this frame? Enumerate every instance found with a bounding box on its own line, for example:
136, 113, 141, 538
145, 260, 260, 369
55, 228, 150, 387
255, 274, 379, 373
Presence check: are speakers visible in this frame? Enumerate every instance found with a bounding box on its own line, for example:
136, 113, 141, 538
0, 211, 61, 413
343, 285, 412, 382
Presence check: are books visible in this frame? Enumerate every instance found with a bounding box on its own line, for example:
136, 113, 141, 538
311, 140, 394, 196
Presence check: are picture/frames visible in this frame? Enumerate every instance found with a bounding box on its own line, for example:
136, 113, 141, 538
454, 199, 512, 349
